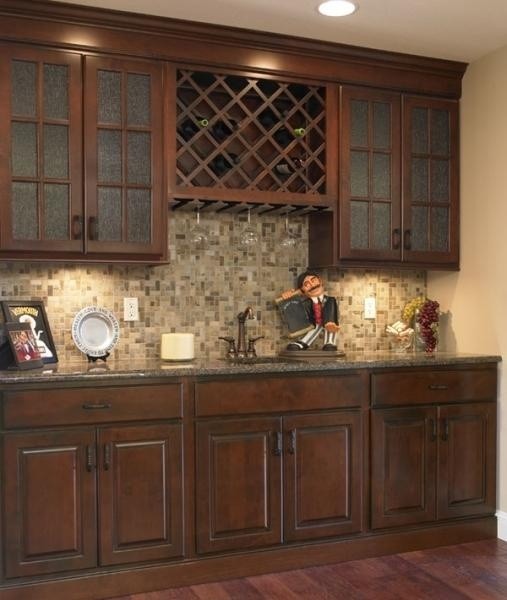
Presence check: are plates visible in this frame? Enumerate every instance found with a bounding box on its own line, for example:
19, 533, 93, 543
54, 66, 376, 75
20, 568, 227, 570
70, 306, 121, 359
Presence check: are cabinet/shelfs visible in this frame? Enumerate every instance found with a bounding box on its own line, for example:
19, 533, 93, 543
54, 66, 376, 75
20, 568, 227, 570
162, 17, 338, 219
1, 0, 176, 266
305, 38, 469, 272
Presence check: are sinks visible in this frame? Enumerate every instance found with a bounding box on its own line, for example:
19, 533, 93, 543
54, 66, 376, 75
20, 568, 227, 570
217, 358, 305, 365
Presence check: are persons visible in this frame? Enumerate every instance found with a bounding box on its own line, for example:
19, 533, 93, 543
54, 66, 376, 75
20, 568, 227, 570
14, 331, 37, 361
281, 271, 342, 351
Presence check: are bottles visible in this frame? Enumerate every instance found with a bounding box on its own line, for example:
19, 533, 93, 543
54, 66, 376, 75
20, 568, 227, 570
181, 115, 209, 136
212, 119, 238, 138
212, 153, 240, 172
275, 126, 307, 145
275, 157, 306, 175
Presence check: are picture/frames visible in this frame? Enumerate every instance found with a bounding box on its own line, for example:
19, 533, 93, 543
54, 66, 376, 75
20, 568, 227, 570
0, 299, 58, 371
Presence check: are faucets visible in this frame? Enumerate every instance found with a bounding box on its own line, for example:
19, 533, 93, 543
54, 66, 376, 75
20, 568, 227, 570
237, 306, 254, 356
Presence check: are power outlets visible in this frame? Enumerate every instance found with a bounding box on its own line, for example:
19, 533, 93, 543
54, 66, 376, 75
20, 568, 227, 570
123, 296, 140, 322
